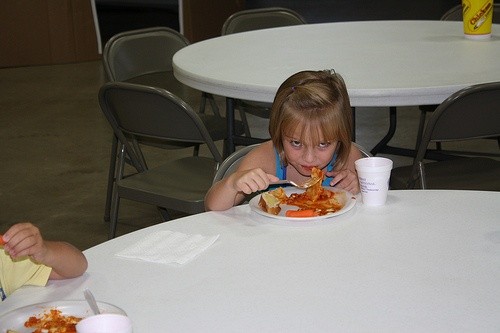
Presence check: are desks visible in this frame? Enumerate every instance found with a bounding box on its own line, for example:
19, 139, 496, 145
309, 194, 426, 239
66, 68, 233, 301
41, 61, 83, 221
0, 188, 500, 333
173, 20, 500, 155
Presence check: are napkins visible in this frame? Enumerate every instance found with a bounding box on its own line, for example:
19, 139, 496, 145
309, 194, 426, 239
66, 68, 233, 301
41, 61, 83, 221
116, 231, 221, 264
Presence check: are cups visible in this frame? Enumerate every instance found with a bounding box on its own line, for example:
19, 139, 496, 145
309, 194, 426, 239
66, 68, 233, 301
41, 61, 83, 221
461, 0, 494, 39
74, 314, 131, 333
355, 155, 394, 206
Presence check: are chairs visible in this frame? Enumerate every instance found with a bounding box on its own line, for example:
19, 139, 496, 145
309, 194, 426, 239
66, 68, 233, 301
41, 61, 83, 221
98, 3, 500, 239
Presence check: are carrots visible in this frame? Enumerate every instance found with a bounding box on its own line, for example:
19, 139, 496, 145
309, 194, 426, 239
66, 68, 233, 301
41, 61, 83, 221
0, 234, 7, 245
286, 209, 314, 217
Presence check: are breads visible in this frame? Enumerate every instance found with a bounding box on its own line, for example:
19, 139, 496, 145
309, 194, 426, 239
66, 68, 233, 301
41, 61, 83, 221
260, 192, 281, 215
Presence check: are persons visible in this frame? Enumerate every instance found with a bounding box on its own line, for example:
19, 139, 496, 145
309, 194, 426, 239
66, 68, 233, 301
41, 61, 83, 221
0, 223, 88, 302
203, 69, 364, 211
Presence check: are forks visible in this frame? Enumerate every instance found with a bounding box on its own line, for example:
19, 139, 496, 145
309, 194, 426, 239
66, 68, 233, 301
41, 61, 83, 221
264, 176, 324, 188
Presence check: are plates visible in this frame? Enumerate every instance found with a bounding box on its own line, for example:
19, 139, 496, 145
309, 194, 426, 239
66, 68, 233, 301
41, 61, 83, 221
0, 300, 129, 333
249, 186, 357, 220
